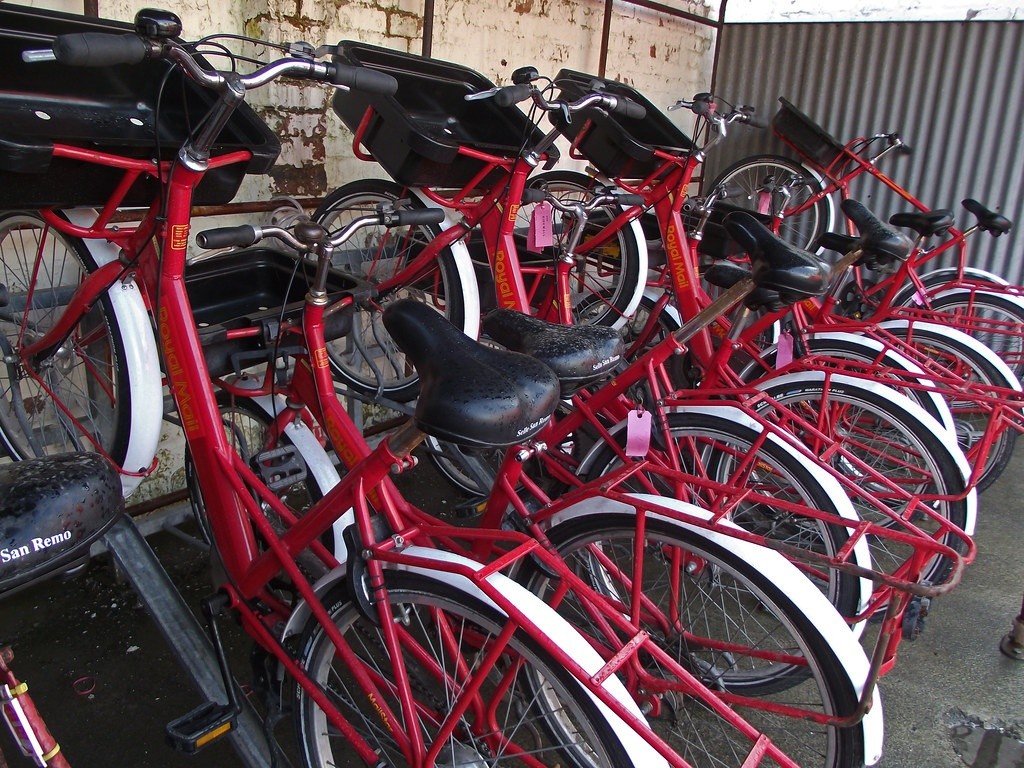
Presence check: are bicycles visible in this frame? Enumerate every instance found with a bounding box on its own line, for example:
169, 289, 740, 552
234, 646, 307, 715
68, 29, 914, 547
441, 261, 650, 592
0, 0, 1024, 767
